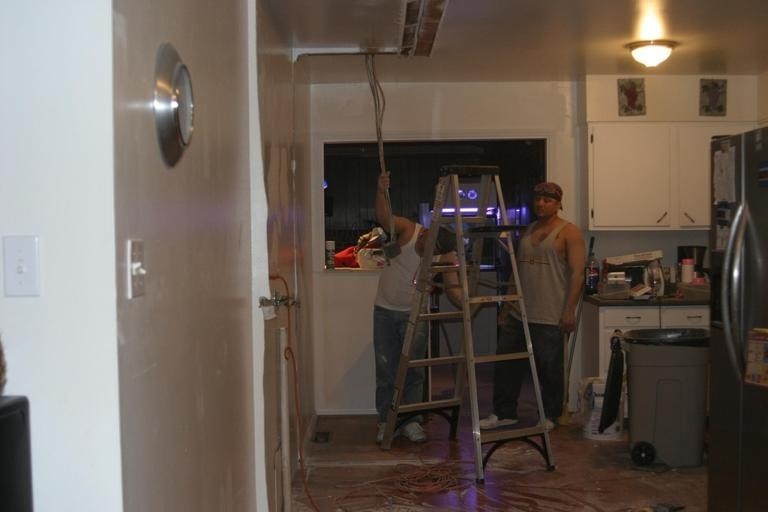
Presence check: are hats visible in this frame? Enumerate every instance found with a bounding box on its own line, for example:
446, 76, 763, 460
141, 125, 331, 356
533, 183, 564, 212
436, 224, 457, 254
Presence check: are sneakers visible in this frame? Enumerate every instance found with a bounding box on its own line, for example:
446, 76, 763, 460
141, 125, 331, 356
376, 420, 399, 443
479, 412, 518, 429
536, 418, 556, 433
402, 418, 427, 444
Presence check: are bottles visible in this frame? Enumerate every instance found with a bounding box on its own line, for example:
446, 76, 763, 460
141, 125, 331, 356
325, 240, 336, 270
681, 258, 694, 284
584, 253, 600, 295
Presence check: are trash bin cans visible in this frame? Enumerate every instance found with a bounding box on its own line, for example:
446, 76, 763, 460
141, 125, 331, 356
598, 328, 711, 468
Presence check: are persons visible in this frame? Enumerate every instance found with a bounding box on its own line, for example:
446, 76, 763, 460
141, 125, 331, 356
480, 182, 582, 430
373, 169, 463, 443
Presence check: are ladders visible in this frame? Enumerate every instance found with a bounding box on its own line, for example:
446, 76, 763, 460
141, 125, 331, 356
381, 166, 558, 486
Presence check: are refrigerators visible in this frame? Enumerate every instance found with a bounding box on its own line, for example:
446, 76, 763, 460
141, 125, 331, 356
707, 126, 767, 512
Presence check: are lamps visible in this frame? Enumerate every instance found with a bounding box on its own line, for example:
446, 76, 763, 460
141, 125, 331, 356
624, 41, 676, 67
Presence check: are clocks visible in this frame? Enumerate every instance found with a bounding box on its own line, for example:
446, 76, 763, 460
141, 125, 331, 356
154, 44, 193, 168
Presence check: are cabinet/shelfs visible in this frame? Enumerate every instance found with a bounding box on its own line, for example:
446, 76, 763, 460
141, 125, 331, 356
582, 288, 710, 420
588, 124, 757, 232
